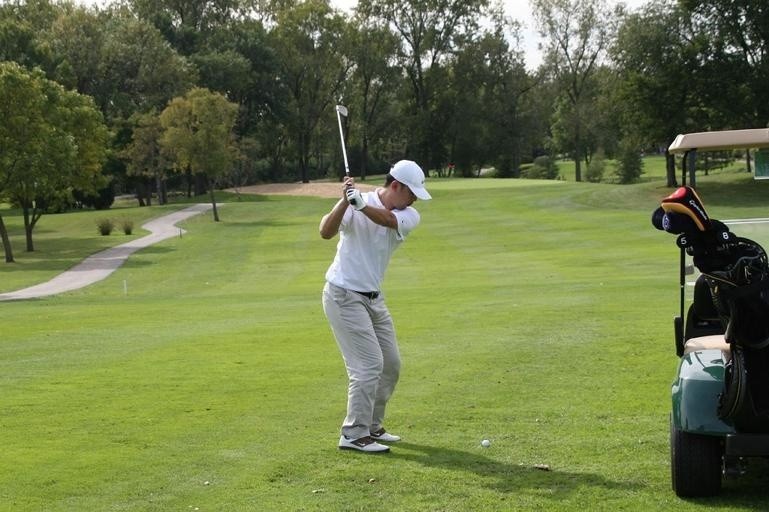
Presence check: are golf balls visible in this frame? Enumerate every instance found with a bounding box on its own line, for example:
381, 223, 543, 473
481, 440, 489, 447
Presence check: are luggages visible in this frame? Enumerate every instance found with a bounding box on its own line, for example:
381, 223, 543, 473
690, 218, 769, 433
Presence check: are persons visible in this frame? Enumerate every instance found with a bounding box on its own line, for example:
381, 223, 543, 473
319, 159, 432, 453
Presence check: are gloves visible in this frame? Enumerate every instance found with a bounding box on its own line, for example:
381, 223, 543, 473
345, 188, 367, 213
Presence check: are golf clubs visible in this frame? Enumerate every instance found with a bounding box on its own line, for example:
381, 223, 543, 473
336, 105, 356, 205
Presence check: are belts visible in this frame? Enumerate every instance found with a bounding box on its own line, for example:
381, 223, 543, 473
351, 289, 380, 300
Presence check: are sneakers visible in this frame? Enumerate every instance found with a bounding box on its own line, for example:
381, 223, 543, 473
369, 427, 402, 442
337, 433, 391, 454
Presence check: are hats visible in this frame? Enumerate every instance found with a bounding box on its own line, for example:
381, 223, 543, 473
389, 159, 433, 201
